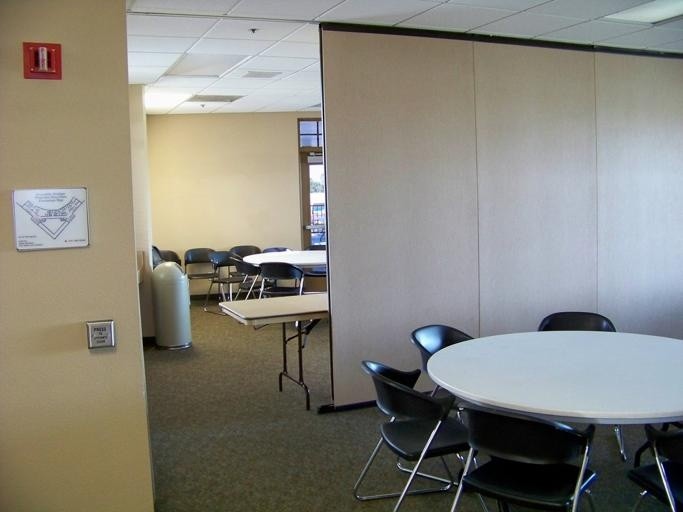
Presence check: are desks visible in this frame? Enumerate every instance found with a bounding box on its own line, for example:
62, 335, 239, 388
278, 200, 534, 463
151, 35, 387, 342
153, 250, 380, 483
218, 292, 330, 411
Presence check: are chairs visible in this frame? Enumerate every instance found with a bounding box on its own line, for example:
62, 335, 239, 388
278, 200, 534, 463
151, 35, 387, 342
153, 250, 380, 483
153, 246, 323, 329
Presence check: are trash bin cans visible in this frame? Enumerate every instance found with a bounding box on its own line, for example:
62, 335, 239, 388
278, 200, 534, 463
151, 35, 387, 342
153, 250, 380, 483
151, 262, 192, 351
